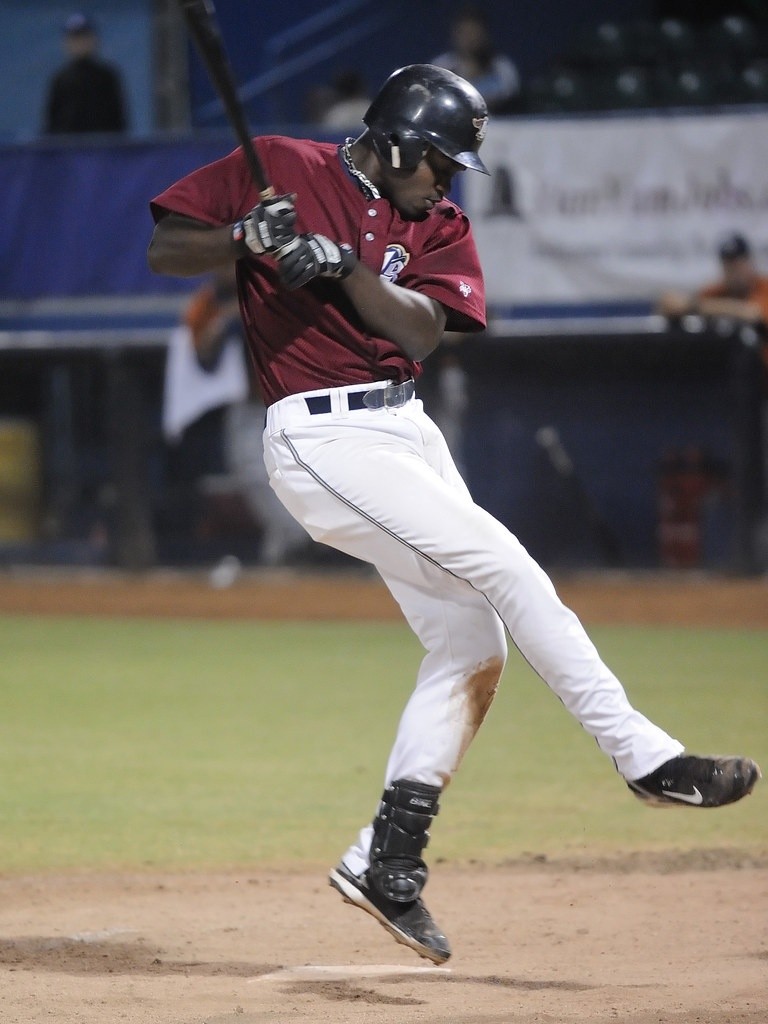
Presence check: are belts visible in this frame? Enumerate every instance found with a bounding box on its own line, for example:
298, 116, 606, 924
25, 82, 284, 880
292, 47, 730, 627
304, 376, 415, 415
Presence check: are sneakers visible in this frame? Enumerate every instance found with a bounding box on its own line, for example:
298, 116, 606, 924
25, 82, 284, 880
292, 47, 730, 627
327, 860, 452, 965
627, 755, 762, 808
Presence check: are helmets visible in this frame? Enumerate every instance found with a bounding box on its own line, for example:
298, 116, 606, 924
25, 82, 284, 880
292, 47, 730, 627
722, 237, 750, 266
361, 64, 493, 177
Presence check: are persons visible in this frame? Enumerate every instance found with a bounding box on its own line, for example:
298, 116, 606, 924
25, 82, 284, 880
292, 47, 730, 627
44, 19, 766, 582
149, 65, 759, 969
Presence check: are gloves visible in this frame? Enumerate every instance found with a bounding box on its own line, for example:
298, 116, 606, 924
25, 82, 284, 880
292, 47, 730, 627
233, 192, 298, 252
277, 231, 357, 291
